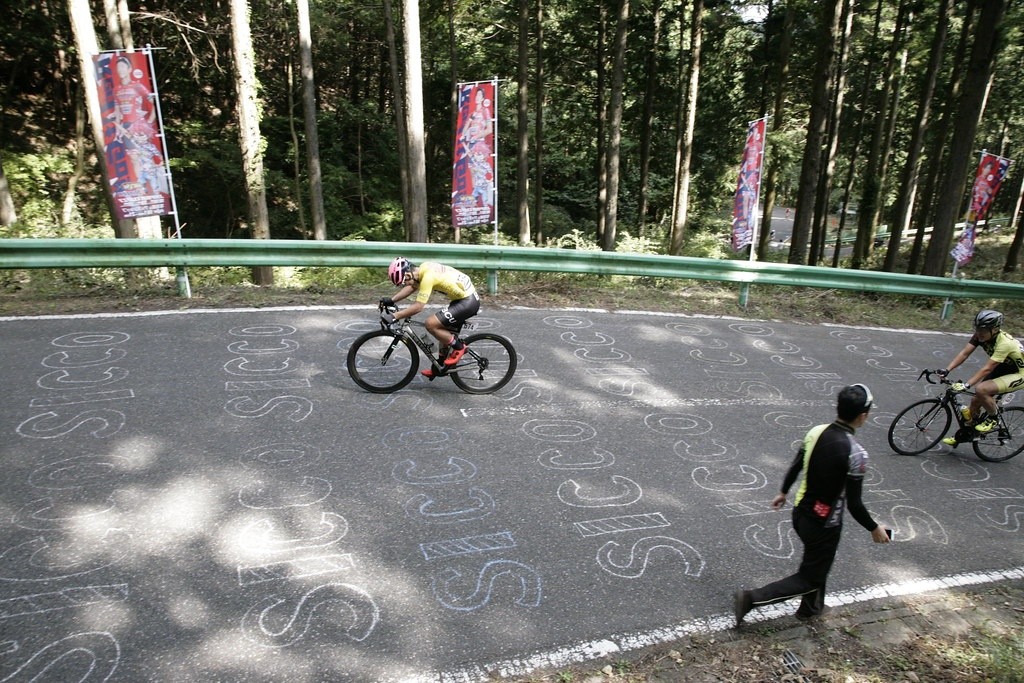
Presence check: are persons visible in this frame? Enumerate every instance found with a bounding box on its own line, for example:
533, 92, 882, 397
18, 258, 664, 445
376, 256, 481, 378
739, 126, 762, 219
970, 161, 993, 214
458, 87, 493, 206
733, 383, 895, 627
112, 56, 162, 195
934, 309, 1024, 448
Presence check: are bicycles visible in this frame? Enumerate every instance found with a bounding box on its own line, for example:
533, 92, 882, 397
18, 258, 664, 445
888, 368, 1024, 469
346, 303, 518, 397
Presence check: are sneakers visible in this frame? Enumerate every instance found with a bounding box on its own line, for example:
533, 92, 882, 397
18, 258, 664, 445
795, 604, 832, 622
943, 436, 957, 445
735, 589, 752, 626
444, 343, 467, 365
974, 415, 1000, 432
421, 370, 433, 377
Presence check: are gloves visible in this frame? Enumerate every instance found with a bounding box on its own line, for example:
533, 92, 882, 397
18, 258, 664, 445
378, 297, 394, 312
952, 383, 969, 392
380, 314, 396, 325
937, 369, 949, 377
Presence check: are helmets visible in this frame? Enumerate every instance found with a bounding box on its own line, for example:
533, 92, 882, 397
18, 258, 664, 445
388, 256, 410, 287
974, 309, 1004, 330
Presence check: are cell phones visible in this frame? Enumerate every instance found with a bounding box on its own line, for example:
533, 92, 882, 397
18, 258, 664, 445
885, 529, 894, 541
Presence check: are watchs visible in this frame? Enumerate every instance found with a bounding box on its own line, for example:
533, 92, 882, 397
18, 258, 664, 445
964, 382, 970, 389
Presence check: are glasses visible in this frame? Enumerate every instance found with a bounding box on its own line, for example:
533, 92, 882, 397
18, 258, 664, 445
400, 275, 406, 285
